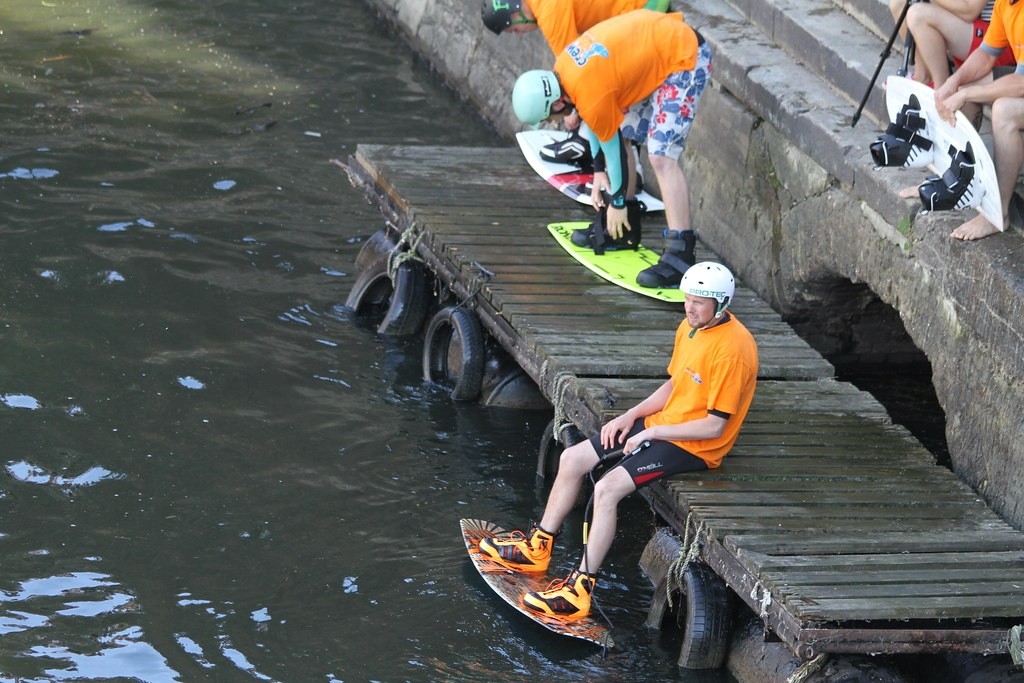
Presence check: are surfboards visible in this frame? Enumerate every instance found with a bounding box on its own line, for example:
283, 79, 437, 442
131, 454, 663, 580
545, 221, 688, 304
460, 517, 615, 648
516, 129, 666, 213
885, 75, 1004, 231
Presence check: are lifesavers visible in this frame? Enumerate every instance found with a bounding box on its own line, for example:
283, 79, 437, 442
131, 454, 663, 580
421, 307, 484, 401
647, 560, 731, 669
345, 248, 431, 336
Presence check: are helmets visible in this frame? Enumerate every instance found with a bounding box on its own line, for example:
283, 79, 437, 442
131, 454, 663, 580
679, 262, 735, 304
511, 69, 561, 132
480, 0, 521, 35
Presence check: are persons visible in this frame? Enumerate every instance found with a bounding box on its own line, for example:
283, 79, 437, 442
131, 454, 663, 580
478, 261, 758, 622
512, 9, 713, 289
889, 0, 1017, 90
481, 0, 671, 196
899, 0, 1024, 241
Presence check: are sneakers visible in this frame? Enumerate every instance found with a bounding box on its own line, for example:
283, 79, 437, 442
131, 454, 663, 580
918, 141, 974, 212
869, 93, 933, 167
636, 228, 697, 288
539, 121, 597, 165
582, 172, 642, 194
478, 527, 554, 571
523, 567, 595, 621
570, 196, 642, 250
